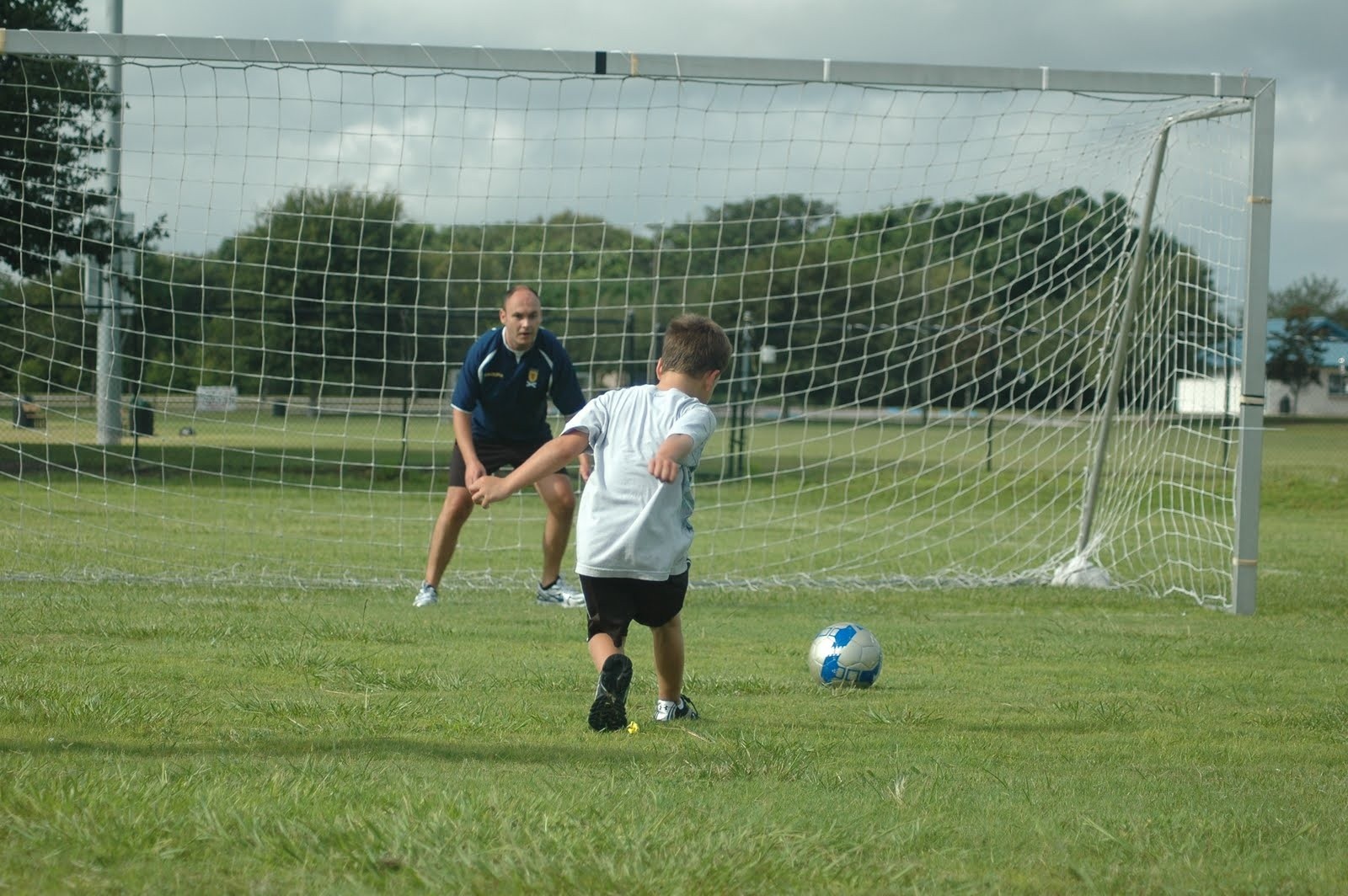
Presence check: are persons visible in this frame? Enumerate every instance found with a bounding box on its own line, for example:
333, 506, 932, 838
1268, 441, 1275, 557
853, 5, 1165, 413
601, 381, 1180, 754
411, 286, 592, 608
468, 314, 733, 730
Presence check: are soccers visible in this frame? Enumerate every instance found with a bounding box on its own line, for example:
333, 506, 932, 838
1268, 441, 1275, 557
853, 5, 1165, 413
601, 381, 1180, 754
809, 620, 882, 687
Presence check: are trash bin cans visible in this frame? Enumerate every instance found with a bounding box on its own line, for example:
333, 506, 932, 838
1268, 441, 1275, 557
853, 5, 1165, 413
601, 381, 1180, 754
129, 398, 153, 435
272, 399, 287, 416
12, 393, 34, 429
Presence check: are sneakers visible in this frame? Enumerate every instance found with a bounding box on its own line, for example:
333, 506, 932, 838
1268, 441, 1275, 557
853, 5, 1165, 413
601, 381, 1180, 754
536, 576, 583, 607
413, 580, 438, 607
589, 654, 633, 731
655, 696, 698, 721
